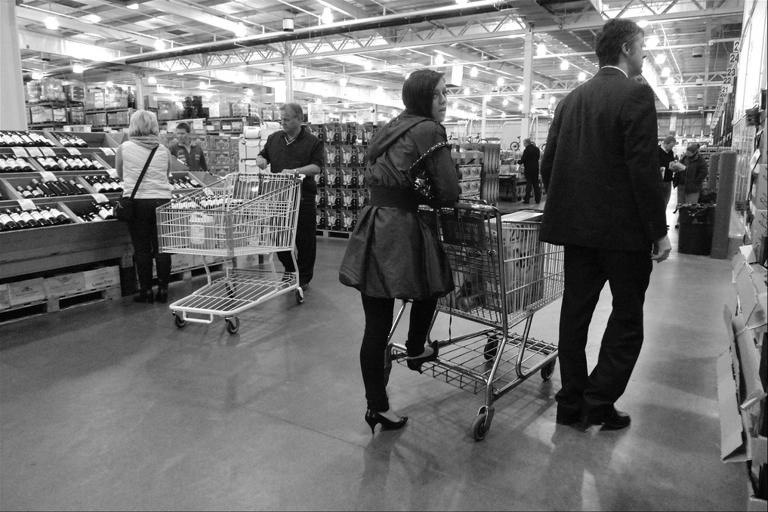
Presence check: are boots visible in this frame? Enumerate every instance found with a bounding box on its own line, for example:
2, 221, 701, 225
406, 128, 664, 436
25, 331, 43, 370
134, 279, 155, 304
156, 275, 168, 303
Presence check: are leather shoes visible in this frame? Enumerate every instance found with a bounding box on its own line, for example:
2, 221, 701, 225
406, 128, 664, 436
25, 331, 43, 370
581, 403, 631, 429
556, 408, 582, 423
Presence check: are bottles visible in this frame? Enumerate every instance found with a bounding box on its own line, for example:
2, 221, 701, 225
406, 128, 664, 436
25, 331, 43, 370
3, 155, 22, 172
101, 176, 121, 192
69, 180, 87, 194
5, 209, 30, 229
7, 131, 28, 146
15, 208, 39, 227
102, 202, 116, 219
173, 179, 183, 189
91, 159, 105, 170
26, 185, 47, 198
52, 181, 70, 195
62, 155, 79, 170
115, 178, 123, 190
85, 176, 106, 193
15, 132, 36, 147
0, 222, 10, 231
0, 132, 15, 146
53, 132, 72, 147
77, 212, 104, 222
185, 176, 201, 188
69, 133, 88, 146
37, 206, 60, 225
29, 210, 51, 226
59, 177, 82, 195
80, 157, 95, 170
60, 133, 79, 147
45, 156, 60, 171
26, 161, 37, 171
0, 137, 7, 146
74, 158, 87, 170
41, 178, 69, 196
173, 192, 244, 209
61, 212, 74, 223
45, 205, 66, 224
32, 179, 56, 196
0, 210, 19, 229
179, 178, 188, 188
17, 186, 33, 198
56, 157, 72, 170
45, 137, 57, 147
93, 176, 114, 192
26, 131, 45, 147
91, 202, 113, 219
168, 181, 176, 189
0, 155, 13, 172
1, 186, 9, 200
37, 156, 54, 171
37, 135, 50, 147
12, 155, 32, 172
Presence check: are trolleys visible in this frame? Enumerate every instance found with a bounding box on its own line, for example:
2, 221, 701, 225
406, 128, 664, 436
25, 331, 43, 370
385, 199, 564, 442
156, 171, 306, 333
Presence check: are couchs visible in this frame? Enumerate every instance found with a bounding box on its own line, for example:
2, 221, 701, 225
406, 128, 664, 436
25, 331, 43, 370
682, 152, 735, 258
697, 153, 720, 209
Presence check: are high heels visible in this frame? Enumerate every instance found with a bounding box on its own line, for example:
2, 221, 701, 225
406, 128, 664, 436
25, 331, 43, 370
407, 340, 439, 374
365, 409, 408, 435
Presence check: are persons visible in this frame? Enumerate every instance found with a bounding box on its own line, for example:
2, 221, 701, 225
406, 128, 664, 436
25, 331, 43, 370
516, 140, 542, 206
255, 103, 325, 292
337, 70, 462, 434
169, 122, 208, 172
539, 20, 672, 431
115, 109, 173, 304
676, 144, 708, 230
657, 136, 676, 231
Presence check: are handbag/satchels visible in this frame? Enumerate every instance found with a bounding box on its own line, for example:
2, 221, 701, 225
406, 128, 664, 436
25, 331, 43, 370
410, 142, 453, 205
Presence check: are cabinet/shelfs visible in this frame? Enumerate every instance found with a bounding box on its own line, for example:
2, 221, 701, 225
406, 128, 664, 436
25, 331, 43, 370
448, 139, 482, 205
92, 85, 139, 127
731, 9, 768, 512
0, 131, 224, 315
497, 149, 549, 199
291, 122, 375, 230
157, 123, 283, 190
22, 79, 89, 128
